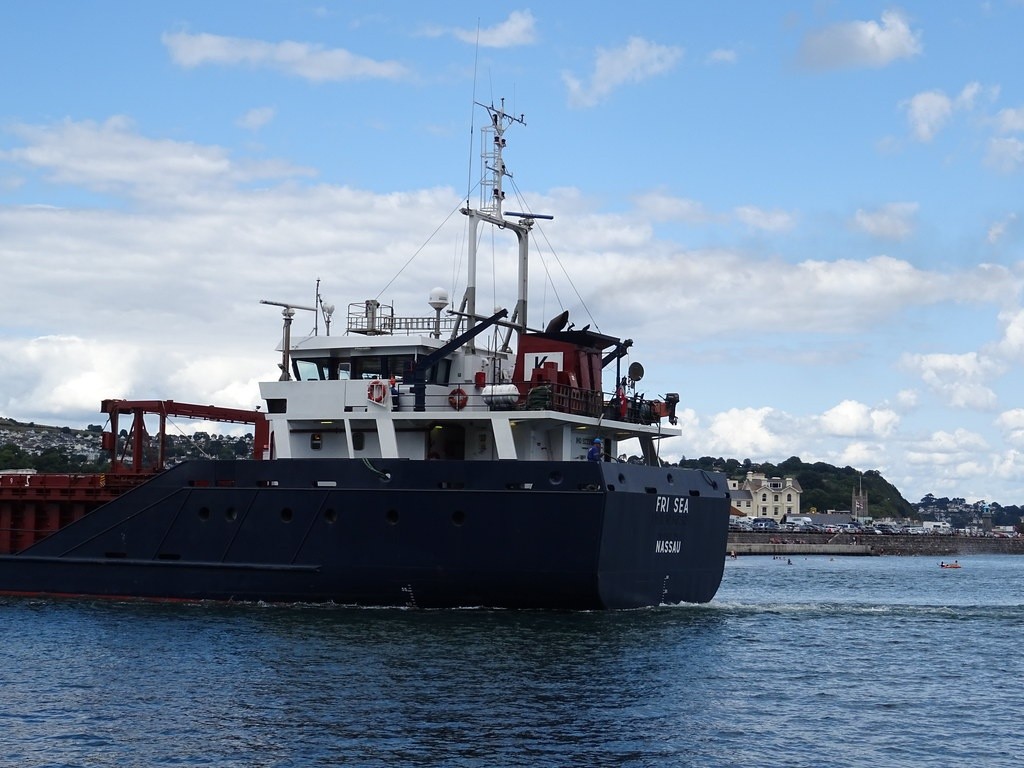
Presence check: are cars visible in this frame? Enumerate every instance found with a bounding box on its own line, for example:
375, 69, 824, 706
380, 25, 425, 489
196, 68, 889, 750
729, 513, 1024, 538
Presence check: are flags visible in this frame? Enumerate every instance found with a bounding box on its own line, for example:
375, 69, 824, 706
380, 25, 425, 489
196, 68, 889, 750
619, 388, 627, 418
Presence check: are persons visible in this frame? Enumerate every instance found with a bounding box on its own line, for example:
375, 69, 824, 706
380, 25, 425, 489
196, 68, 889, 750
941, 561, 943, 565
587, 438, 605, 462
730, 549, 734, 558
734, 551, 737, 558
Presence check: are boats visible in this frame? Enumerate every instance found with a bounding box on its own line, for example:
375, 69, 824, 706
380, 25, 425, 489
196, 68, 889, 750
0, 93, 731, 619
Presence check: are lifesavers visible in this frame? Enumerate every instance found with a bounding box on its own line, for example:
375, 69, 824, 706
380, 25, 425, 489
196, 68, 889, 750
448, 388, 467, 410
367, 380, 386, 401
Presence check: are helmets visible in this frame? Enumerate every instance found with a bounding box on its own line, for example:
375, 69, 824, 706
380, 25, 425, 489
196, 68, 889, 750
593, 438, 602, 445
